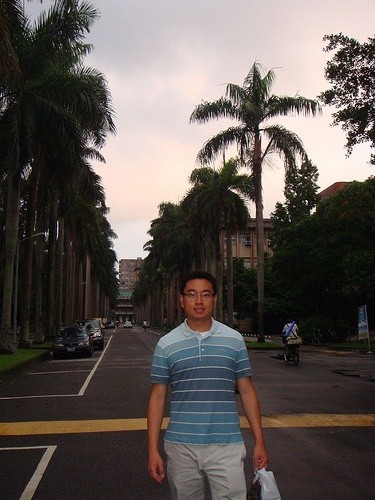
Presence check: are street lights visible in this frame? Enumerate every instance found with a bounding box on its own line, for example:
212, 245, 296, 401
13, 232, 45, 349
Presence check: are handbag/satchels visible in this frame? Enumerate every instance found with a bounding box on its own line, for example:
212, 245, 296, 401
253, 467, 282, 500
247, 476, 262, 500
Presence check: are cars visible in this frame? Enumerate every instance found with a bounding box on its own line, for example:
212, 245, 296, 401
76, 318, 120, 348
53, 325, 94, 356
123, 321, 133, 328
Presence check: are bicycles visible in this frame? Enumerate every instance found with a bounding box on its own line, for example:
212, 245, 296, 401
281, 336, 300, 365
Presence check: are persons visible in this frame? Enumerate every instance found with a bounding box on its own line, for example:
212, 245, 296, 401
147, 271, 268, 500
143, 319, 147, 332
280, 317, 299, 358
74, 318, 82, 326
116, 319, 119, 327
61, 321, 64, 326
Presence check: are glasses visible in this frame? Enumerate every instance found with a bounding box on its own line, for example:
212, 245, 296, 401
182, 291, 214, 299
